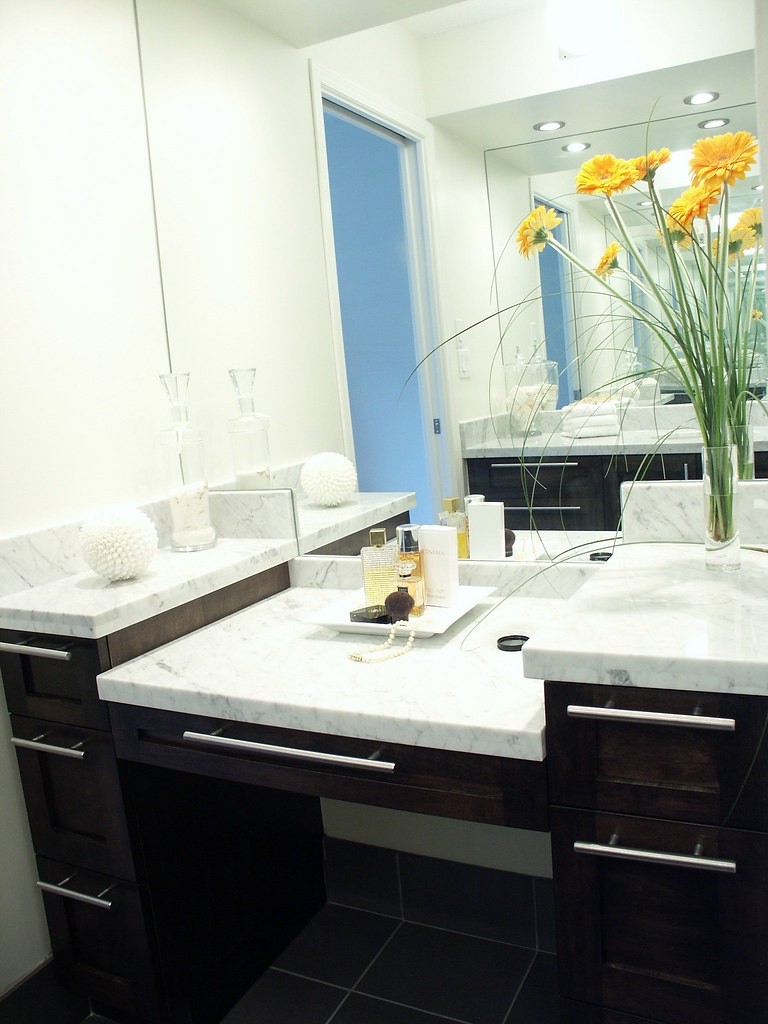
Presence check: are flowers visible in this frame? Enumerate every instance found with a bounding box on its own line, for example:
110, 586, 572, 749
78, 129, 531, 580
595, 208, 762, 425
516, 129, 768, 539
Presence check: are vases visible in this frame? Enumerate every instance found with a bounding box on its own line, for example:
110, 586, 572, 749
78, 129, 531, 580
728, 426, 754, 480
700, 445, 741, 570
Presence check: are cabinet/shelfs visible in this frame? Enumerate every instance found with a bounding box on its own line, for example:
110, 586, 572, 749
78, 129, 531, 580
467, 452, 768, 531
0, 628, 768, 1024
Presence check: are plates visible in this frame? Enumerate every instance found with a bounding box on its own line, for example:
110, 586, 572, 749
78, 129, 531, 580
291, 583, 497, 639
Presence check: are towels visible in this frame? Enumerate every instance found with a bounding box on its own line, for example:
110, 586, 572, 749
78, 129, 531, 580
561, 399, 620, 438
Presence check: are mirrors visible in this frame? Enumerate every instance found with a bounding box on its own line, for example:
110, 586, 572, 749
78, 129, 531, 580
483, 103, 768, 410
700, 271, 768, 348
603, 195, 768, 390
657, 245, 768, 369
136, 0, 768, 563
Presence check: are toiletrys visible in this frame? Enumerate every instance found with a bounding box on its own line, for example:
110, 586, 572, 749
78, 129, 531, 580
438, 494, 508, 563
360, 523, 461, 618
